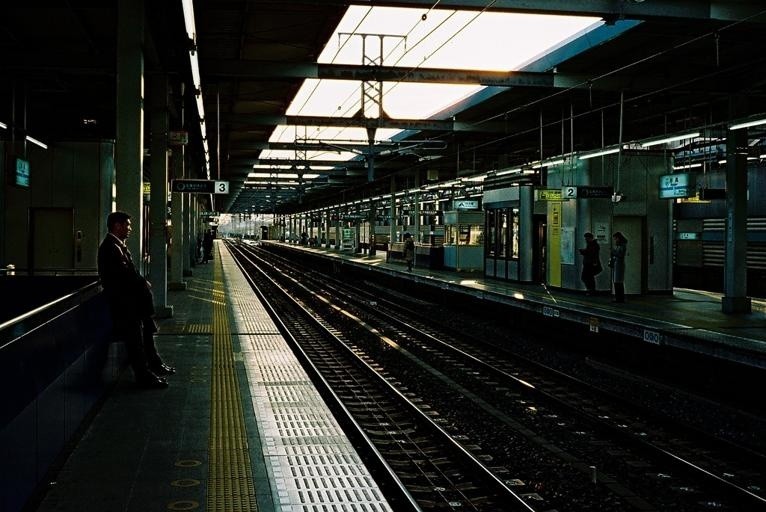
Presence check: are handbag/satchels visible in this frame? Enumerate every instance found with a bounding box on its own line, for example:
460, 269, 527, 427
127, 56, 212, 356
608, 256, 616, 269
593, 258, 603, 276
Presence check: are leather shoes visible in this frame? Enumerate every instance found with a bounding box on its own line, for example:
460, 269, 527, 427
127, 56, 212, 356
141, 363, 175, 390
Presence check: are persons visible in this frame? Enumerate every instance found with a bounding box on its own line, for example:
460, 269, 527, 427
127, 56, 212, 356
200, 228, 215, 264
578, 232, 603, 296
140, 322, 176, 377
608, 232, 628, 303
403, 232, 414, 273
97, 210, 177, 389
